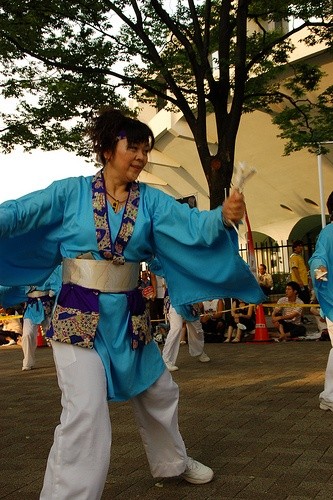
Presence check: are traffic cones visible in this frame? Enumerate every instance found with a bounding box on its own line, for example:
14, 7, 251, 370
247, 303, 275, 343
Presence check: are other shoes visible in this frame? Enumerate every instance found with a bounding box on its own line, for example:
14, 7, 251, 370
166, 364, 179, 372
22, 365, 32, 371
231, 337, 241, 344
223, 338, 231, 343
7, 340, 16, 345
318, 398, 332, 412
197, 351, 211, 363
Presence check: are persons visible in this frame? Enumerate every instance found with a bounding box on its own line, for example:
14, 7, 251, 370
0, 190, 333, 419
0, 109, 252, 500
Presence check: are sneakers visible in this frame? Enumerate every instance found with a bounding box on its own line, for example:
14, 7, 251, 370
177, 455, 215, 486
